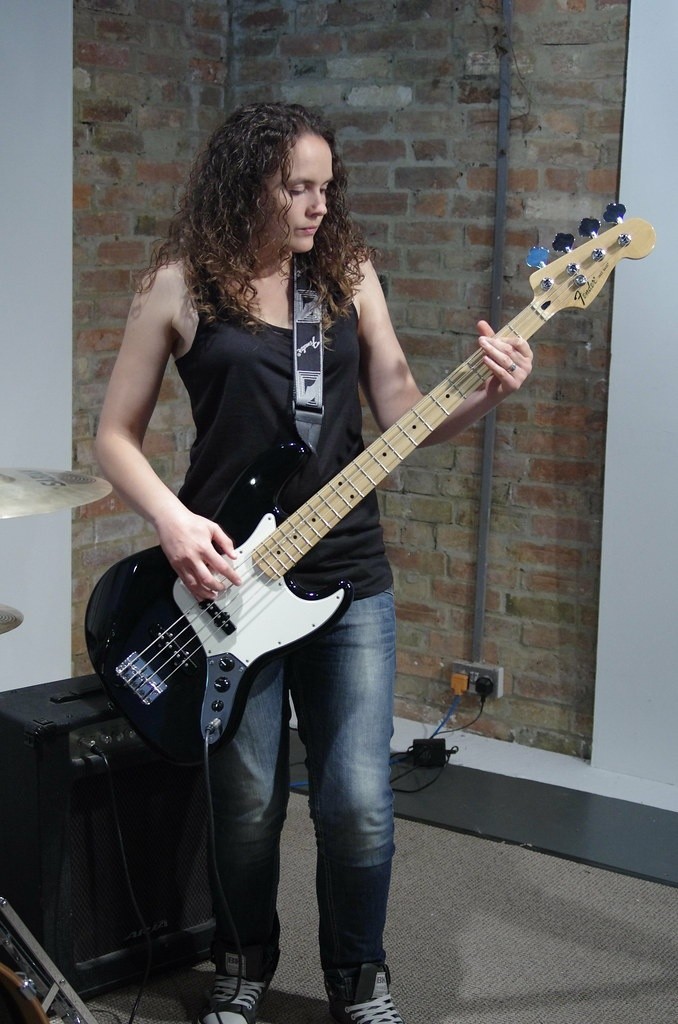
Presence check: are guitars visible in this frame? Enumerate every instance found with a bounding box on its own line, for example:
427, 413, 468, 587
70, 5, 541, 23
81, 199, 660, 759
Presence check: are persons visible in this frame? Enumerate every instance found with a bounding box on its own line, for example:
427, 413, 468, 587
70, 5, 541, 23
94, 101, 533, 1024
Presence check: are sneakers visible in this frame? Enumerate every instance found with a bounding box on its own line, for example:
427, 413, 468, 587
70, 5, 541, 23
323, 960, 400, 1024
196, 944, 280, 1024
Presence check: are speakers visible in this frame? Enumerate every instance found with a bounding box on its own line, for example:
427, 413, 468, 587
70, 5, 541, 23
0, 671, 216, 1002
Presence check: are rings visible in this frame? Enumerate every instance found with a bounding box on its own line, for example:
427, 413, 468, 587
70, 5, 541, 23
505, 363, 516, 373
